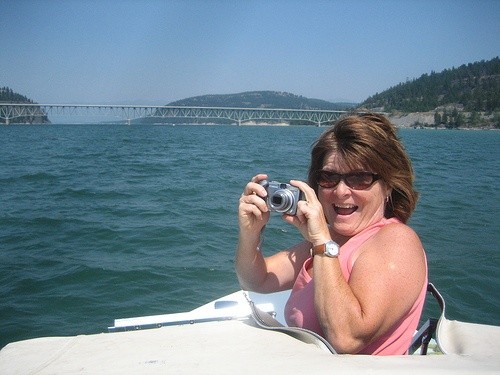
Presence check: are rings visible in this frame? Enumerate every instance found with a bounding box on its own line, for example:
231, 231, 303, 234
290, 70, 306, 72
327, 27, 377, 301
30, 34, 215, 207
305, 200, 309, 206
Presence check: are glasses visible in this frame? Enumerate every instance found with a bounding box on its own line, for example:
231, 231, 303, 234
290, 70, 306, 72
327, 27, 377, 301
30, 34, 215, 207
314, 170, 381, 190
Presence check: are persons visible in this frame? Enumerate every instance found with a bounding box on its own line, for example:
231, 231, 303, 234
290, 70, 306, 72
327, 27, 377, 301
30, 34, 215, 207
236, 112, 428, 356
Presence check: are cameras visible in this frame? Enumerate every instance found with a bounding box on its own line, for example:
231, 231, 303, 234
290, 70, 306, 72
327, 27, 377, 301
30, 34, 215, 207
256, 180, 302, 216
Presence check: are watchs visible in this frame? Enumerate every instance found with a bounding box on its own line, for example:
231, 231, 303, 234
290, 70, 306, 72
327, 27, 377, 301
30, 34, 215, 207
310, 240, 340, 258
